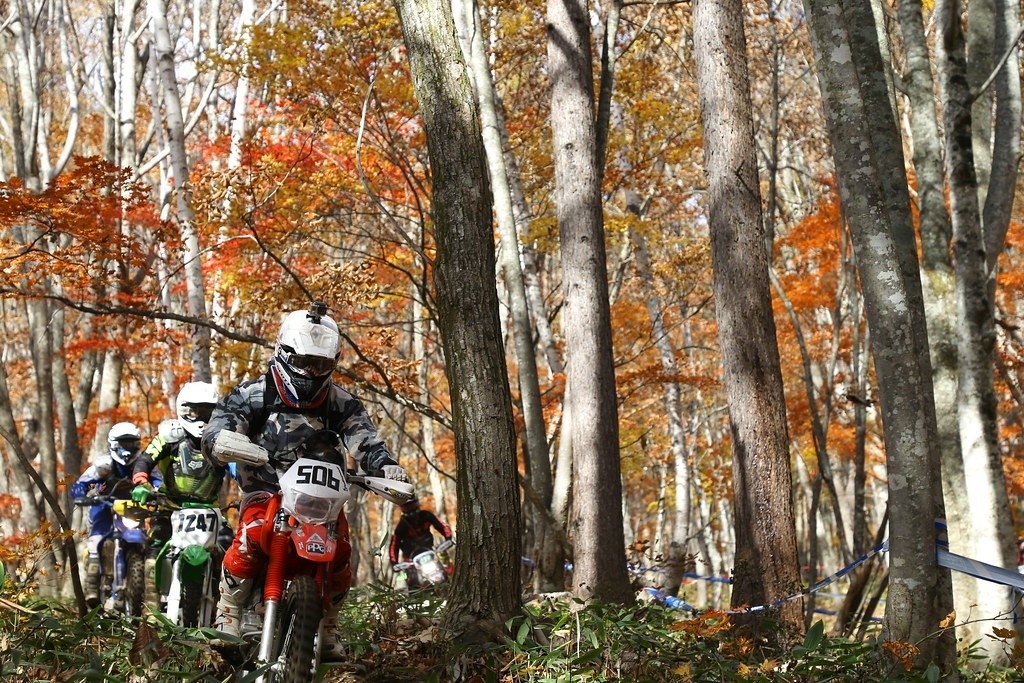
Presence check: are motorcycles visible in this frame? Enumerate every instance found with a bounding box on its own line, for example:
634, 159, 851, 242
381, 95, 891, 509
73, 491, 170, 630
130, 486, 241, 636
390, 539, 453, 604
210, 433, 418, 683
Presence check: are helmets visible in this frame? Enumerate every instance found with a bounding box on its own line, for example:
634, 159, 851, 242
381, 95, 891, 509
106, 422, 142, 466
176, 381, 221, 439
271, 308, 342, 410
400, 494, 421, 519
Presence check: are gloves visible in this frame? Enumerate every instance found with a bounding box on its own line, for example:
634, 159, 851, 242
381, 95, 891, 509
381, 464, 406, 483
131, 483, 153, 505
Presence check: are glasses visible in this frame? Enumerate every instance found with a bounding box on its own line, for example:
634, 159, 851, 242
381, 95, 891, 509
275, 339, 341, 375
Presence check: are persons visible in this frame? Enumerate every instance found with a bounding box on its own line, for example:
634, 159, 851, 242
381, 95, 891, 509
72, 421, 164, 616
132, 381, 234, 628
201, 309, 412, 667
389, 495, 453, 597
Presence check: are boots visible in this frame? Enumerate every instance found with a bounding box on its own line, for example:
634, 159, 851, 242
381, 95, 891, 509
84, 562, 100, 602
319, 609, 349, 662
216, 562, 252, 640
140, 558, 159, 628
